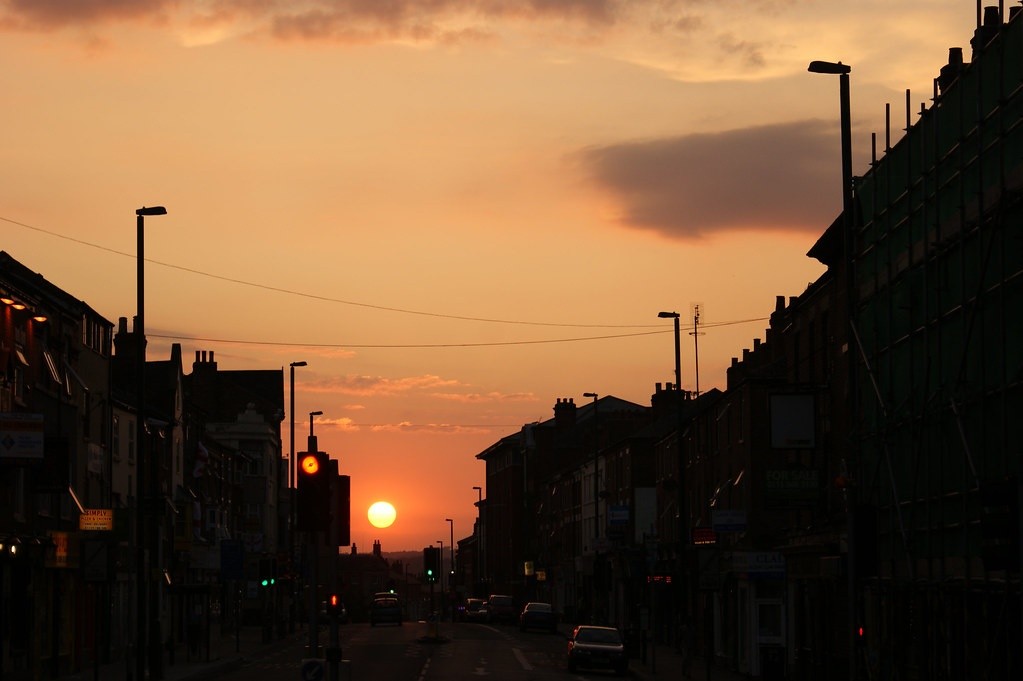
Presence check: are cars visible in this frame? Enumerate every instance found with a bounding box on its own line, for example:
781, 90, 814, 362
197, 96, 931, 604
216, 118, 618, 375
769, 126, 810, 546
487, 595, 519, 622
520, 602, 557, 635
370, 592, 402, 626
477, 602, 488, 621
464, 598, 483, 621
566, 625, 628, 674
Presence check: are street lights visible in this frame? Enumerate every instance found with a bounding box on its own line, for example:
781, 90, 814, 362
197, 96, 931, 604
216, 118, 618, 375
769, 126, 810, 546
288, 361, 308, 632
583, 393, 598, 584
472, 487, 482, 503
445, 519, 454, 570
437, 541, 444, 623
134, 205, 168, 678
310, 410, 324, 437
657, 311, 690, 558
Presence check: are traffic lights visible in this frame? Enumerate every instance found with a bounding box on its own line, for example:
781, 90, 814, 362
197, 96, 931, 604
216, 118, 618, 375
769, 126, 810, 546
423, 548, 439, 578
257, 559, 276, 591
387, 586, 397, 593
297, 452, 330, 531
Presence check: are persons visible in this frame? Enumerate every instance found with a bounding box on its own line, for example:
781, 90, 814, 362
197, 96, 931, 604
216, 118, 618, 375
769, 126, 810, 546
677, 614, 698, 681
